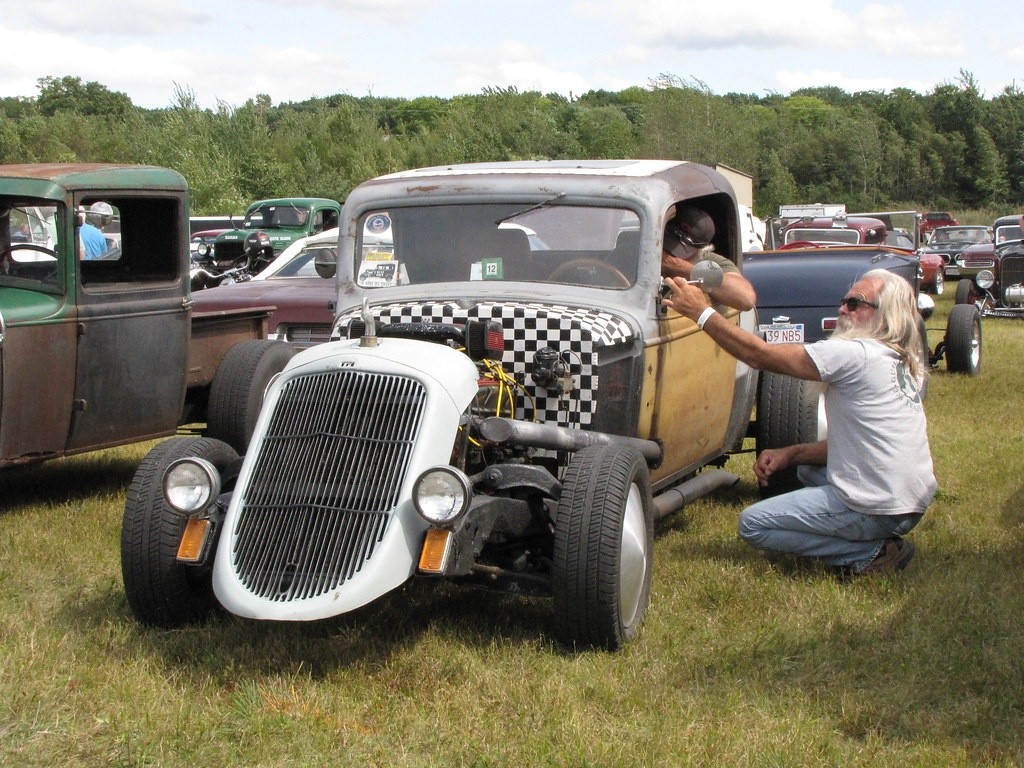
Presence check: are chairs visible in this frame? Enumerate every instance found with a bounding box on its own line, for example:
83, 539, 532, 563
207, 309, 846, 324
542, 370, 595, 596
441, 228, 547, 283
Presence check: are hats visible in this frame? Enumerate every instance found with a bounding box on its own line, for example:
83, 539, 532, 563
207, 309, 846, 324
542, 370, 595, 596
662, 206, 715, 260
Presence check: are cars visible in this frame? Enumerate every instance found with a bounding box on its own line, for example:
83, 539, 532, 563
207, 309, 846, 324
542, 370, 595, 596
738, 202, 1024, 386
17, 195, 555, 352
117, 160, 832, 661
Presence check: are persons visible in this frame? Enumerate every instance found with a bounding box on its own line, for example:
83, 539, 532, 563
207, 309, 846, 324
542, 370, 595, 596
33, 206, 85, 260
661, 268, 937, 584
77, 206, 108, 260
601, 209, 757, 312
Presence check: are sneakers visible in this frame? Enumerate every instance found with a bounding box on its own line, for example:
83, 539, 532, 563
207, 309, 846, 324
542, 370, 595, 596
859, 536, 915, 577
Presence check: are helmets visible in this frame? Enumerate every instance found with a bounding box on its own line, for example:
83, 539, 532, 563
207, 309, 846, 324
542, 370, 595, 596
244, 231, 273, 261
87, 201, 113, 227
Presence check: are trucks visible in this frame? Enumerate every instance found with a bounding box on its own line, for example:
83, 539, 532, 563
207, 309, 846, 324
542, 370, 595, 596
0, 159, 297, 469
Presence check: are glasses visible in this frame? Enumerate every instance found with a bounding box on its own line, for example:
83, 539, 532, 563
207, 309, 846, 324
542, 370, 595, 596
840, 297, 879, 312
670, 223, 709, 246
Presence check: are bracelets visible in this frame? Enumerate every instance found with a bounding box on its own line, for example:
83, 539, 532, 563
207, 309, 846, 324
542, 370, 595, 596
696, 307, 716, 330
